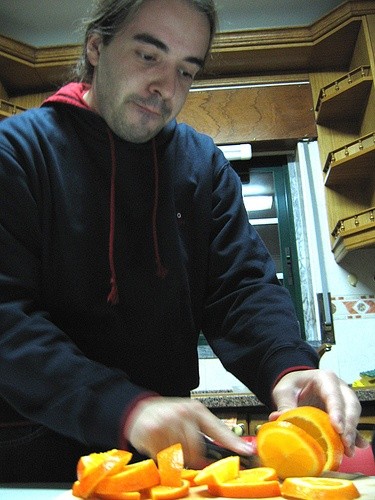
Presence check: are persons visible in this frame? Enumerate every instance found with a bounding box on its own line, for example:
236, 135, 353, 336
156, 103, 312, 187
1, 0, 363, 481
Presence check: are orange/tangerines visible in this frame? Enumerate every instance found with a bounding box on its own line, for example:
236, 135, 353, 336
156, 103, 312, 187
71, 405, 361, 500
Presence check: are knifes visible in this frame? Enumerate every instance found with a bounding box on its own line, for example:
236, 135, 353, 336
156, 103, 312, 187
203, 436, 262, 471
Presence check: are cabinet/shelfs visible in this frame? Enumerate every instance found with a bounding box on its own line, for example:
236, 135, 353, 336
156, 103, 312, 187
307, 15, 375, 265
